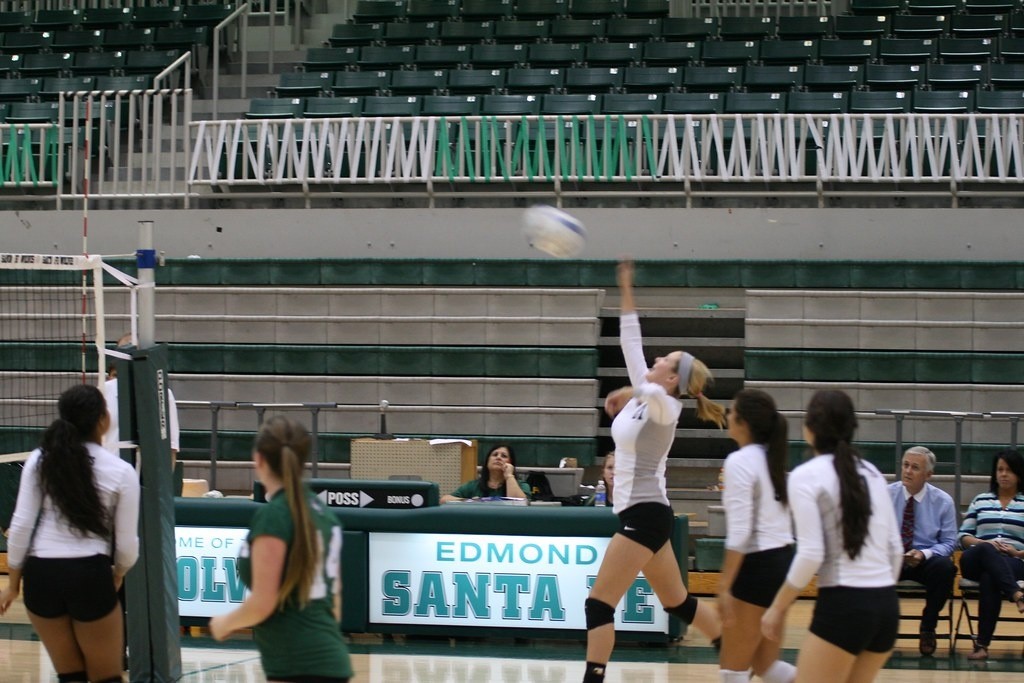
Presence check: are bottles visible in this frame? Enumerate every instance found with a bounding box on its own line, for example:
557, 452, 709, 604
595, 480, 606, 506
718, 469, 725, 490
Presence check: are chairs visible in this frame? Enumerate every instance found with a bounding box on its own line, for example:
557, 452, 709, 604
0, 0, 1024, 208
898, 551, 1024, 655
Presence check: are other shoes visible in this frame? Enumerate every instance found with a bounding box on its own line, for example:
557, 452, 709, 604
967, 645, 987, 659
1016, 594, 1024, 612
919, 623, 936, 655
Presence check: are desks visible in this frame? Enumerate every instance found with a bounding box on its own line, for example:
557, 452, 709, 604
169, 498, 690, 650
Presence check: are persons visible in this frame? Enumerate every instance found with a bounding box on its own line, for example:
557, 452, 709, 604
886, 447, 958, 654
958, 450, 1024, 658
718, 389, 797, 683
582, 253, 725, 683
97, 334, 180, 473
0, 383, 140, 683
761, 390, 904, 683
439, 444, 531, 506
210, 414, 355, 683
564, 450, 615, 507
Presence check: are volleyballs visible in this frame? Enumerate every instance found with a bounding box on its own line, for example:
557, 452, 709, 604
523, 204, 589, 261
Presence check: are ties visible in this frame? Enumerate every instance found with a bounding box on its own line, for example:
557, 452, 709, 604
901, 497, 914, 552
